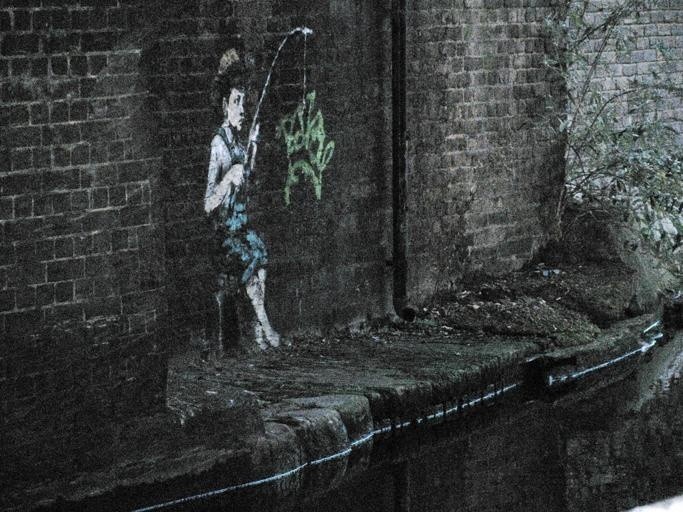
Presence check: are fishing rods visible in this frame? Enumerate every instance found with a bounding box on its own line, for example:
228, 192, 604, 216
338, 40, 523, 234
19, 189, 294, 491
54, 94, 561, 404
227, 26, 311, 209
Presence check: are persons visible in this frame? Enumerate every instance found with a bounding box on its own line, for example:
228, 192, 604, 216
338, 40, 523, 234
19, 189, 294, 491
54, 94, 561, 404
202, 51, 287, 353
